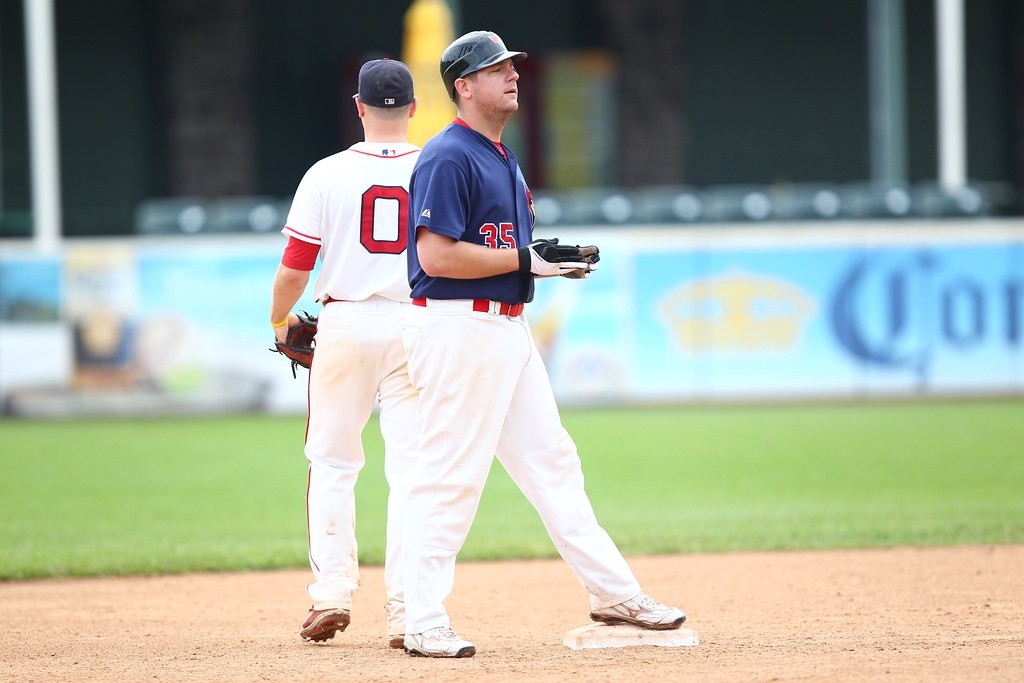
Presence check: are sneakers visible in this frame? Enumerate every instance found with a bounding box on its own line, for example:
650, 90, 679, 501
387, 634, 404, 650
590, 594, 687, 629
299, 605, 351, 646
404, 626, 477, 658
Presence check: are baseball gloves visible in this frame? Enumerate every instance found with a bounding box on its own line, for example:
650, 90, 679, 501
269, 309, 318, 380
518, 237, 600, 294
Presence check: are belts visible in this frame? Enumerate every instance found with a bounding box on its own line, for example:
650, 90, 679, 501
411, 297, 525, 317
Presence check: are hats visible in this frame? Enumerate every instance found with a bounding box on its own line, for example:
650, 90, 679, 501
358, 59, 414, 109
439, 29, 527, 99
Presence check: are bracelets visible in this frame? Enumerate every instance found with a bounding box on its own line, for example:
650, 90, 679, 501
272, 316, 287, 328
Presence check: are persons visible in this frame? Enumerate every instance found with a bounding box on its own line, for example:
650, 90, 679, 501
403, 31, 685, 657
273, 58, 422, 648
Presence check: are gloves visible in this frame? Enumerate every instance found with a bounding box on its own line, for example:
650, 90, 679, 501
517, 236, 601, 280
